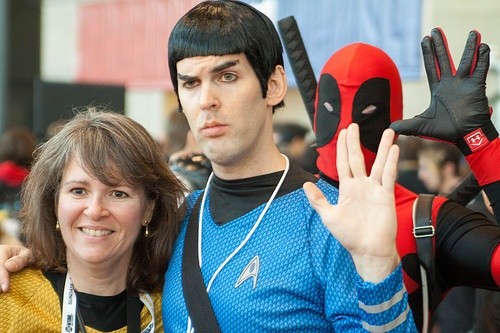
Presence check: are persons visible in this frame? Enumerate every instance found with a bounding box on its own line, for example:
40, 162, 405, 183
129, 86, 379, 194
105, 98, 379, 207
0, 111, 213, 333
0, 0, 419, 333
312, 26, 500, 333
273, 121, 500, 333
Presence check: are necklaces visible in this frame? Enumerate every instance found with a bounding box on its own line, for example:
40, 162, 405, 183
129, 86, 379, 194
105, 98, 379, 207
61, 268, 155, 333
186, 153, 289, 333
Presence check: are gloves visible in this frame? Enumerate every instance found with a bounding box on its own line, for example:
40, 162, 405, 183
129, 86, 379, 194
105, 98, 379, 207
390, 27, 499, 157
